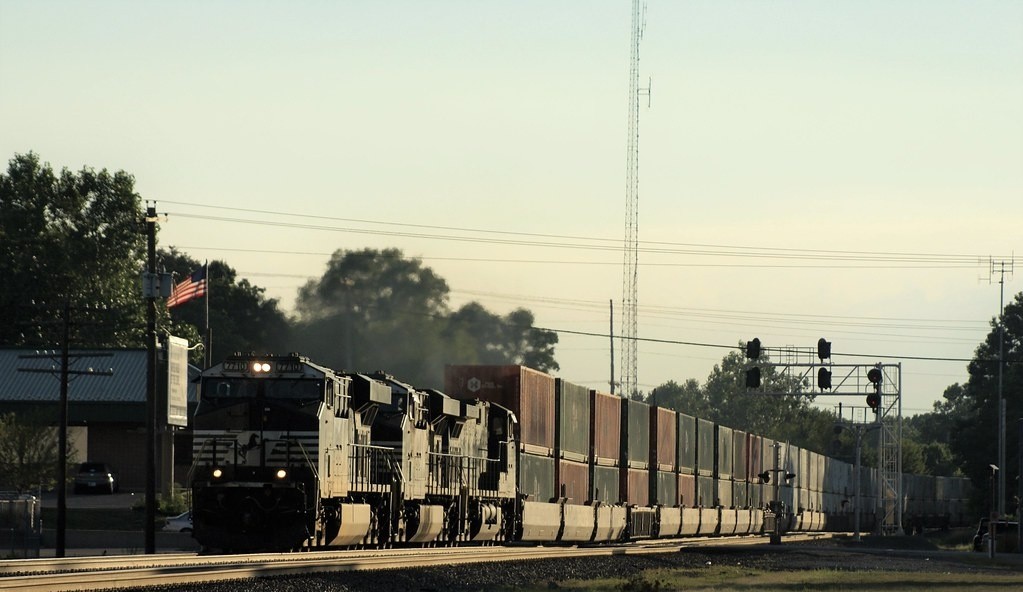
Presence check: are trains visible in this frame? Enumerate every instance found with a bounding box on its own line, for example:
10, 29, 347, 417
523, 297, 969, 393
191, 353, 991, 554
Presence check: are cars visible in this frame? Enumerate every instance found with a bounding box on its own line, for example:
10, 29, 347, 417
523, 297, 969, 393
74, 462, 116, 495
163, 510, 194, 532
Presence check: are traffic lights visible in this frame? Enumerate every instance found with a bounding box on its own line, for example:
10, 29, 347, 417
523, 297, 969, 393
866, 393, 881, 407
747, 367, 760, 389
818, 339, 832, 359
747, 338, 761, 360
867, 369, 883, 383
819, 367, 832, 389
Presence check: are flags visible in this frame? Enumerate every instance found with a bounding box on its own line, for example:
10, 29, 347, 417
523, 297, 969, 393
166, 263, 207, 308
